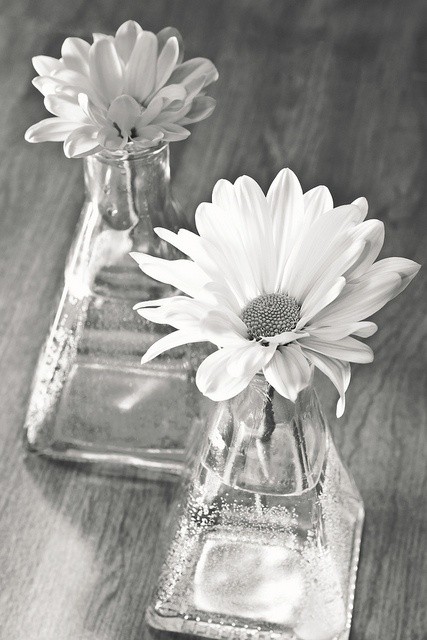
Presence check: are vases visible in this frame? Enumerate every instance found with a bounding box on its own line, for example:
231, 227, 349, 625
28, 143, 213, 484
146, 371, 363, 640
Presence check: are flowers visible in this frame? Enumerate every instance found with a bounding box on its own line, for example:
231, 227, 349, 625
132, 167, 421, 477
25, 22, 219, 212
